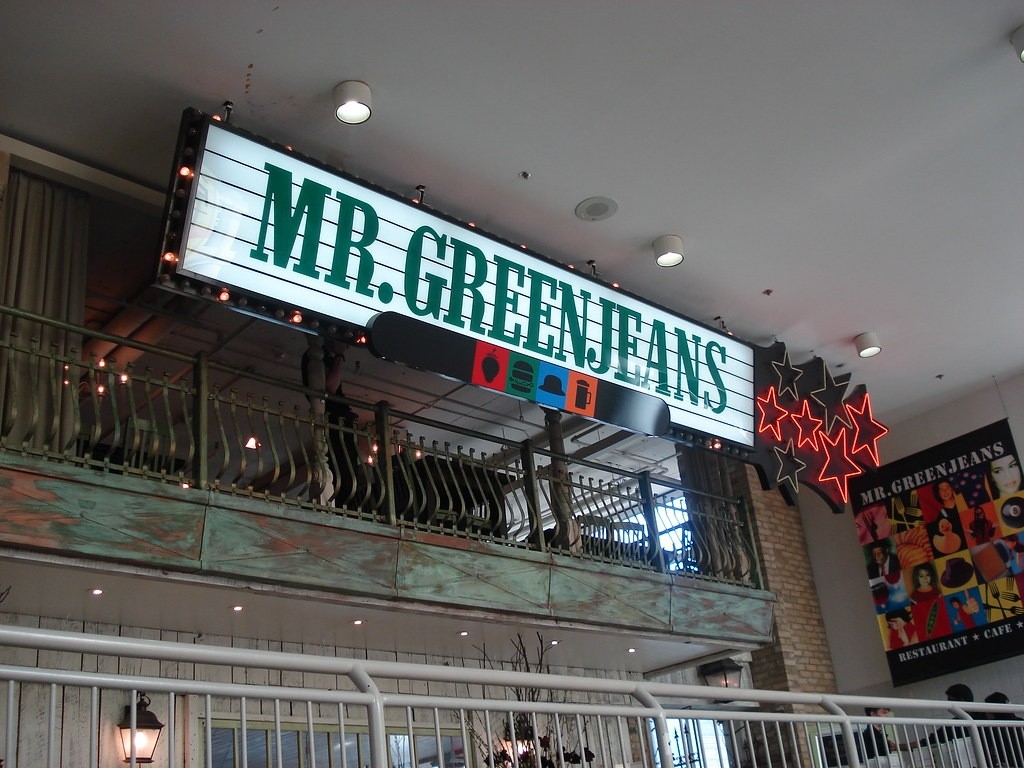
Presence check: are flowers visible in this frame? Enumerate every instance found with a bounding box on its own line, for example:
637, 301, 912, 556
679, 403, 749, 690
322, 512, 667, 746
450, 628, 596, 768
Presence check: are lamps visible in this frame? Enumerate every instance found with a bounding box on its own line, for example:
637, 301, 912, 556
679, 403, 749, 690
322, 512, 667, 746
116, 690, 165, 762
651, 234, 686, 266
699, 656, 744, 706
854, 331, 883, 357
332, 80, 374, 127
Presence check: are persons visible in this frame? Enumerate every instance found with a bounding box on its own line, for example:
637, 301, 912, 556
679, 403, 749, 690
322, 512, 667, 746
936, 481, 958, 520
968, 506, 997, 545
863, 708, 891, 759
986, 454, 1021, 501
868, 541, 901, 579
910, 562, 941, 605
887, 684, 1024, 768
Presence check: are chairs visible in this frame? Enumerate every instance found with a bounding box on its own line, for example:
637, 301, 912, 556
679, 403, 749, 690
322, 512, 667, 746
122, 416, 176, 473
575, 515, 647, 565
371, 449, 415, 526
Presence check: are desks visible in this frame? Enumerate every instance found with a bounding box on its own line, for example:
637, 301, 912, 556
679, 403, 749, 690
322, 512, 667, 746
73, 440, 188, 476
520, 527, 669, 570
411, 454, 516, 546
